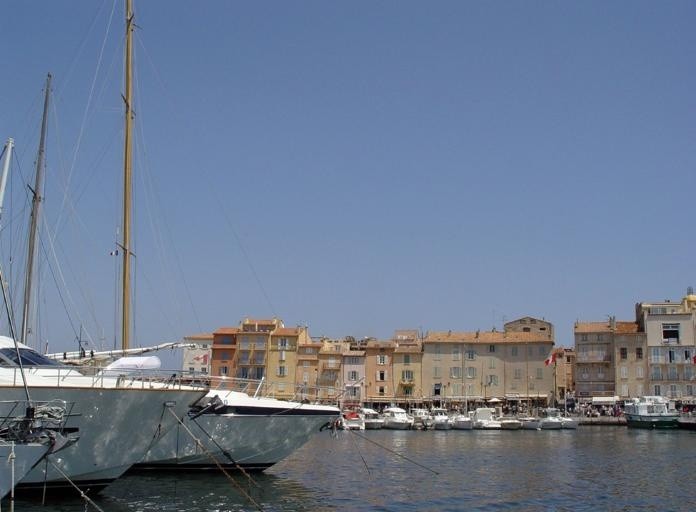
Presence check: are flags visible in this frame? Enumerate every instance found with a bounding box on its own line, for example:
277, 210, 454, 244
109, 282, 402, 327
544, 352, 556, 368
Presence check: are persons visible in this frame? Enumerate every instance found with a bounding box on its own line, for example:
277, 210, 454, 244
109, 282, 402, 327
409, 397, 696, 416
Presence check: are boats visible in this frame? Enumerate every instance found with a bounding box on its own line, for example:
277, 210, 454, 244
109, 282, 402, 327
624, 396, 683, 428
0, 397, 82, 503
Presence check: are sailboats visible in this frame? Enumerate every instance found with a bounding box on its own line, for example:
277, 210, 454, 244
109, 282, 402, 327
333, 341, 578, 430
0, 71, 209, 493
44, 1, 344, 476
